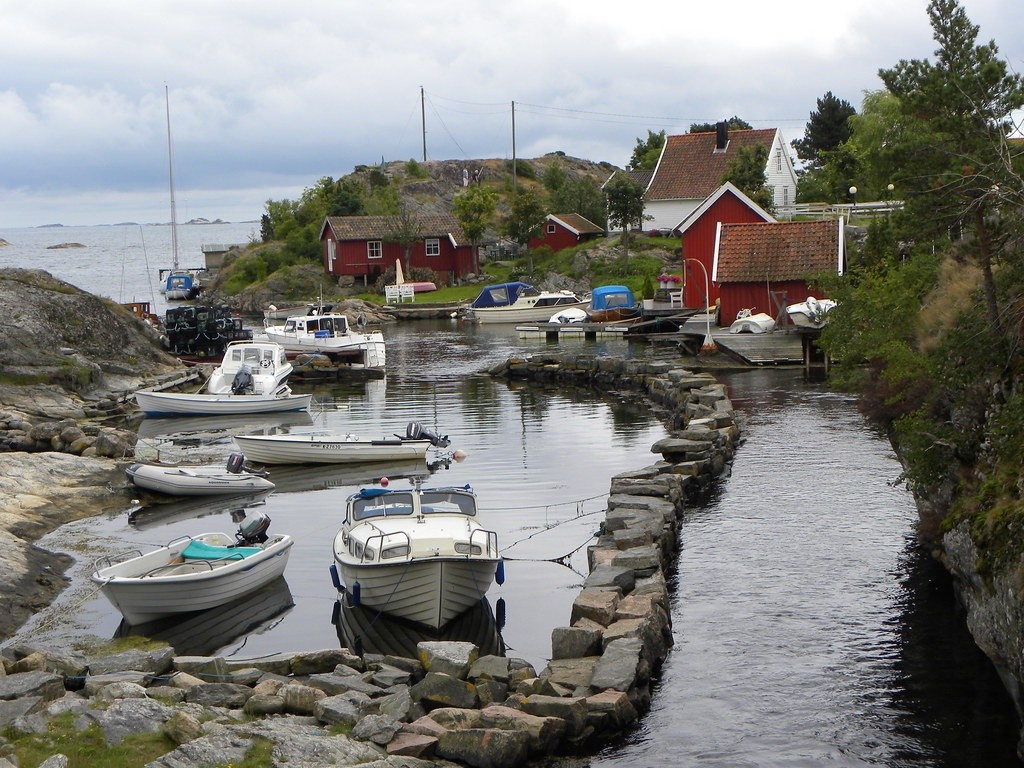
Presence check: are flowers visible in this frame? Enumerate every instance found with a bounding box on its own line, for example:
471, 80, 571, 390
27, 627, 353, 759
656, 275, 682, 283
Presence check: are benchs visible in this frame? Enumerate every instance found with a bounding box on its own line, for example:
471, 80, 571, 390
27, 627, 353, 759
181, 540, 261, 567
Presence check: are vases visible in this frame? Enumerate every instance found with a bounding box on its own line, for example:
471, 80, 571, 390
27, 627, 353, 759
659, 281, 675, 288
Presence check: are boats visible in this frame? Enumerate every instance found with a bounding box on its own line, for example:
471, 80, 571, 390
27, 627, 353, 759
134, 384, 312, 421
329, 474, 505, 631
785, 294, 840, 330
586, 284, 642, 325
127, 486, 277, 532
91, 510, 293, 627
165, 273, 193, 300
264, 314, 386, 368
265, 458, 429, 495
125, 453, 275, 494
549, 307, 587, 324
209, 338, 294, 395
332, 587, 507, 661
464, 282, 592, 324
234, 422, 451, 466
137, 410, 315, 441
110, 575, 296, 656
727, 312, 776, 335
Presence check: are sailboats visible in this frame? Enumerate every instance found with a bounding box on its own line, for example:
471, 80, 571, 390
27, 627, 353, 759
158, 83, 202, 292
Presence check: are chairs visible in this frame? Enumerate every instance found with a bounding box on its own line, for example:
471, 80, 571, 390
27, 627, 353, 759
669, 288, 683, 308
385, 284, 416, 303
